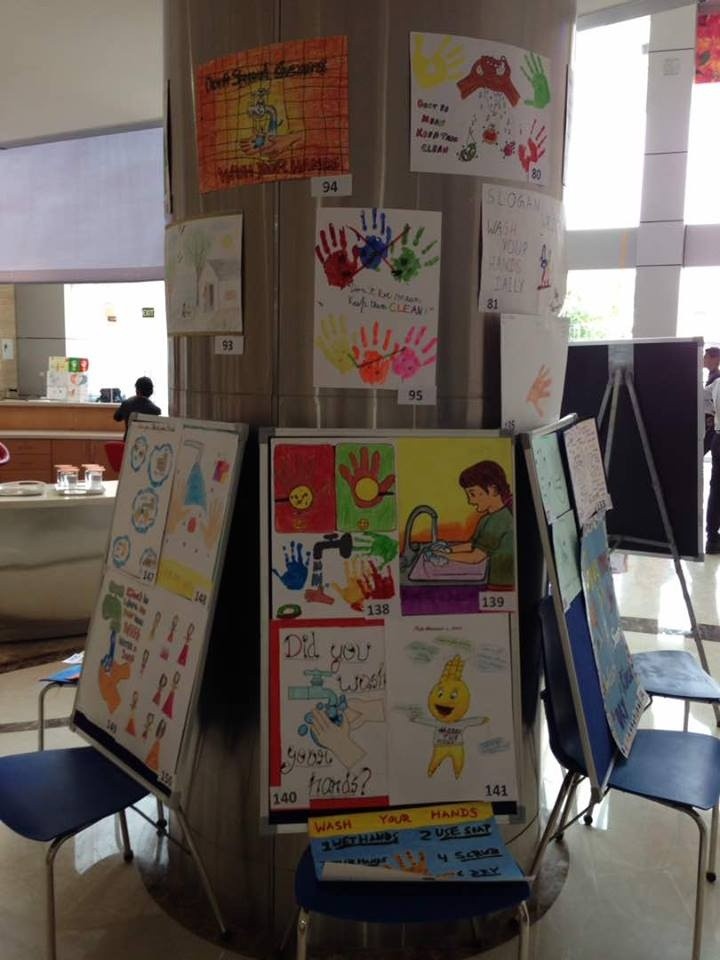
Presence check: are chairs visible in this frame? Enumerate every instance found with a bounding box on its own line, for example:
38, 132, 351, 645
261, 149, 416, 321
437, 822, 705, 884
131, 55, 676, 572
0, 666, 232, 960
298, 594, 720, 960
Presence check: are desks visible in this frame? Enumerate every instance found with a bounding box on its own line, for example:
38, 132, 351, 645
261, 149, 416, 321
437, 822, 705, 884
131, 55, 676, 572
0, 478, 118, 641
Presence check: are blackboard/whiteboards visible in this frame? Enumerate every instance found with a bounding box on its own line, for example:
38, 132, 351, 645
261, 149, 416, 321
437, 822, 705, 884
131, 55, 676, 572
561, 336, 705, 562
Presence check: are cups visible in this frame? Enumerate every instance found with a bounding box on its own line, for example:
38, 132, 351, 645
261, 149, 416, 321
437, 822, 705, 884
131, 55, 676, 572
56, 464, 102, 492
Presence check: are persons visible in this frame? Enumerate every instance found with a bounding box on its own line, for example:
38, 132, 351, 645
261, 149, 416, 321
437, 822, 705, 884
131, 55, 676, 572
704, 347, 720, 554
113, 376, 161, 441
